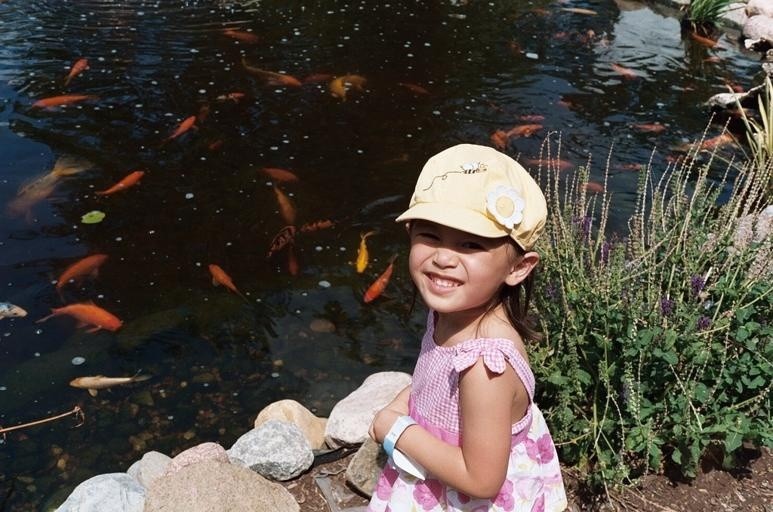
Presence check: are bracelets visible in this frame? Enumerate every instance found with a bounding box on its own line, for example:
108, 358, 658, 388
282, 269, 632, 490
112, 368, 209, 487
381, 415, 431, 480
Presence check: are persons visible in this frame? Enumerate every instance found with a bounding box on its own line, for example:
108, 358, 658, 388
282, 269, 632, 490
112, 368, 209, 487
367, 142, 570, 512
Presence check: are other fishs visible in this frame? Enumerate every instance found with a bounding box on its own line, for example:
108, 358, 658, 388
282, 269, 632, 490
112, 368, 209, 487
222, 25, 257, 44
690, 32, 728, 54
168, 114, 199, 139
206, 262, 241, 298
399, 83, 428, 95
258, 166, 404, 304
559, 7, 597, 16
217, 58, 371, 103
490, 112, 734, 195
65, 368, 157, 398
606, 58, 638, 85
1, 298, 28, 324
33, 250, 124, 338
9, 153, 146, 215
32, 57, 96, 109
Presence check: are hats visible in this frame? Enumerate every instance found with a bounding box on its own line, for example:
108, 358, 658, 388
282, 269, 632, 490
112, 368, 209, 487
394, 139, 548, 253
